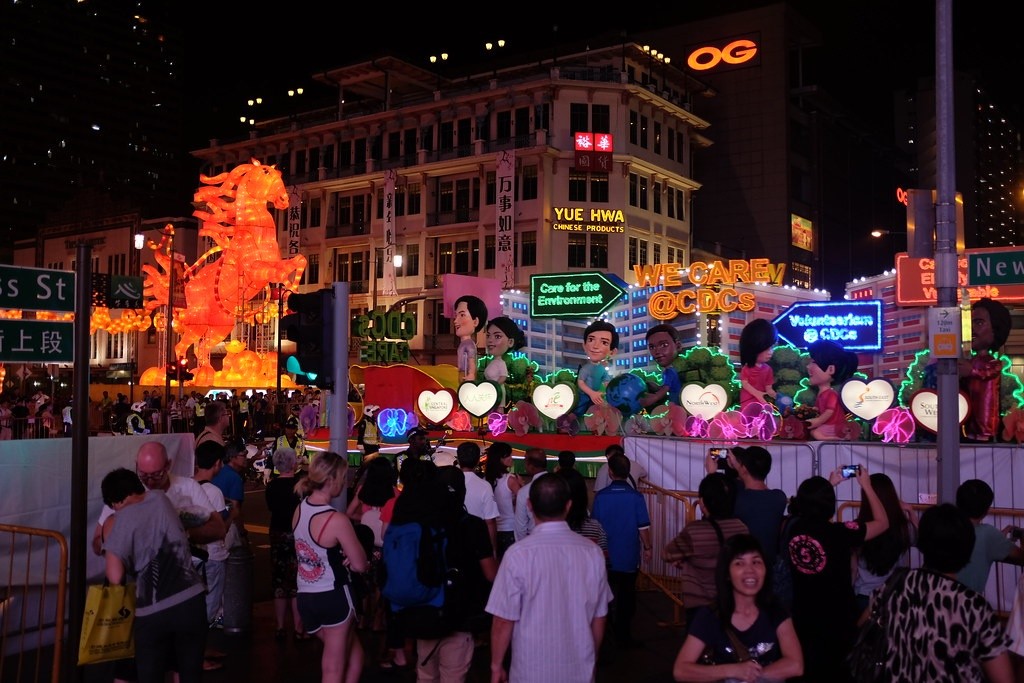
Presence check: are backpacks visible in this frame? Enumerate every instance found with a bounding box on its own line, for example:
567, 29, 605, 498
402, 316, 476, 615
382, 512, 472, 612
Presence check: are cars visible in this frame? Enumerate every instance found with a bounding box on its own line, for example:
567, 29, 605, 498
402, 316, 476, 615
105, 363, 132, 379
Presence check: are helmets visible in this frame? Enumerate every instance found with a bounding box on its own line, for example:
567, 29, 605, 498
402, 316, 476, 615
363, 405, 380, 418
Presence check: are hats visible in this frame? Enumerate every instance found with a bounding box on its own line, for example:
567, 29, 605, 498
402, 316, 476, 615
406, 428, 428, 441
285, 417, 298, 427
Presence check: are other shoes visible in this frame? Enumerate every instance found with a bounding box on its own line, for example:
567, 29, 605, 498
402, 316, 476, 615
292, 630, 315, 643
377, 645, 412, 673
274, 628, 288, 637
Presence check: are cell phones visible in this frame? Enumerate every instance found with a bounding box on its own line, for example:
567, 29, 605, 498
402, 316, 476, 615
710, 448, 728, 460
841, 465, 860, 478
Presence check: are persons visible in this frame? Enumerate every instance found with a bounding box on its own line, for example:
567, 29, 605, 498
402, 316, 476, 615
660, 445, 1024, 683
265, 417, 653, 683
574, 321, 619, 417
959, 298, 1011, 440
0, 384, 320, 442
638, 324, 683, 408
804, 339, 859, 441
357, 405, 382, 459
452, 295, 524, 414
739, 318, 777, 411
91, 400, 272, 683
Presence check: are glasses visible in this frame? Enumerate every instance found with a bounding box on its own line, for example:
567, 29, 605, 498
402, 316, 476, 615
135, 465, 168, 482
225, 412, 230, 416
237, 450, 248, 458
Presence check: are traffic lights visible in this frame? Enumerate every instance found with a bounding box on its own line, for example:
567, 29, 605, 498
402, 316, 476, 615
282, 293, 322, 384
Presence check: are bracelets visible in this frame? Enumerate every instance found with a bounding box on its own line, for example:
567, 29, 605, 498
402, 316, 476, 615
643, 545, 652, 550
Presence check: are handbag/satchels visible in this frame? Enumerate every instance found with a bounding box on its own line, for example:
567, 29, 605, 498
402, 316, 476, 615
78, 575, 136, 665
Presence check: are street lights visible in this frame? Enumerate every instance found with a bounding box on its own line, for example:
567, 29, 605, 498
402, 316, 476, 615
34, 374, 68, 414
134, 223, 176, 431
373, 240, 404, 311
